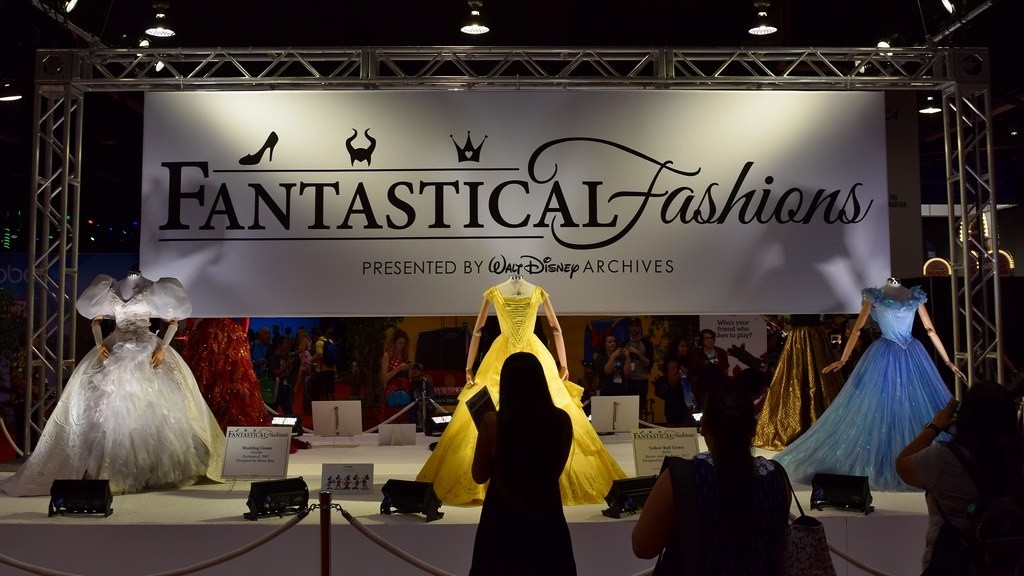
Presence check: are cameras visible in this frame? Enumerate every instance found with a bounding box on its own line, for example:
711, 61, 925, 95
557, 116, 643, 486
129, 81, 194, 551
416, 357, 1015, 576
953, 400, 961, 417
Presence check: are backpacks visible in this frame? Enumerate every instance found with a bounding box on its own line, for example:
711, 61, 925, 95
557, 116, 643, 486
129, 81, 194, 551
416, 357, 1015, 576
317, 338, 341, 365
919, 441, 1024, 576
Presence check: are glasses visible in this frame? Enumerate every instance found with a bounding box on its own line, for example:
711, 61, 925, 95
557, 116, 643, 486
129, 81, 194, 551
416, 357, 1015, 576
704, 336, 713, 342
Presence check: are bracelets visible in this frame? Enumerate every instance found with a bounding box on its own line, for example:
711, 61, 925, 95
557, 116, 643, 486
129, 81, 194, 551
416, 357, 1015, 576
1013, 369, 1018, 376
922, 421, 941, 435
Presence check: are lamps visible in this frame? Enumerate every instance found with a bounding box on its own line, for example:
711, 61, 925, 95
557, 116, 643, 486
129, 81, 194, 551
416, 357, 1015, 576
601, 474, 658, 519
747, 0, 778, 35
918, 95, 942, 114
380, 479, 444, 523
0, 83, 23, 101
244, 475, 309, 522
49, 478, 113, 518
460, 0, 490, 35
876, 33, 905, 56
810, 472, 875, 516
423, 413, 453, 436
144, 0, 176, 38
941, 0, 967, 22
271, 416, 304, 438
54, 0, 78, 12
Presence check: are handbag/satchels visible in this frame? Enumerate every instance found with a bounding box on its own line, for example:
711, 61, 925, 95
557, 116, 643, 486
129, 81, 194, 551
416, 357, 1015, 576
654, 376, 672, 398
386, 390, 411, 407
767, 459, 836, 576
257, 364, 280, 404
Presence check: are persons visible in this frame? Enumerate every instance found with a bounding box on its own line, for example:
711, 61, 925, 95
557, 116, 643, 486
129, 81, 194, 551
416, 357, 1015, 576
895, 379, 1024, 576
246, 324, 413, 426
465, 352, 578, 576
588, 316, 788, 429
752, 277, 966, 493
0, 269, 311, 497
960, 326, 1024, 386
416, 274, 628, 505
632, 387, 792, 576
